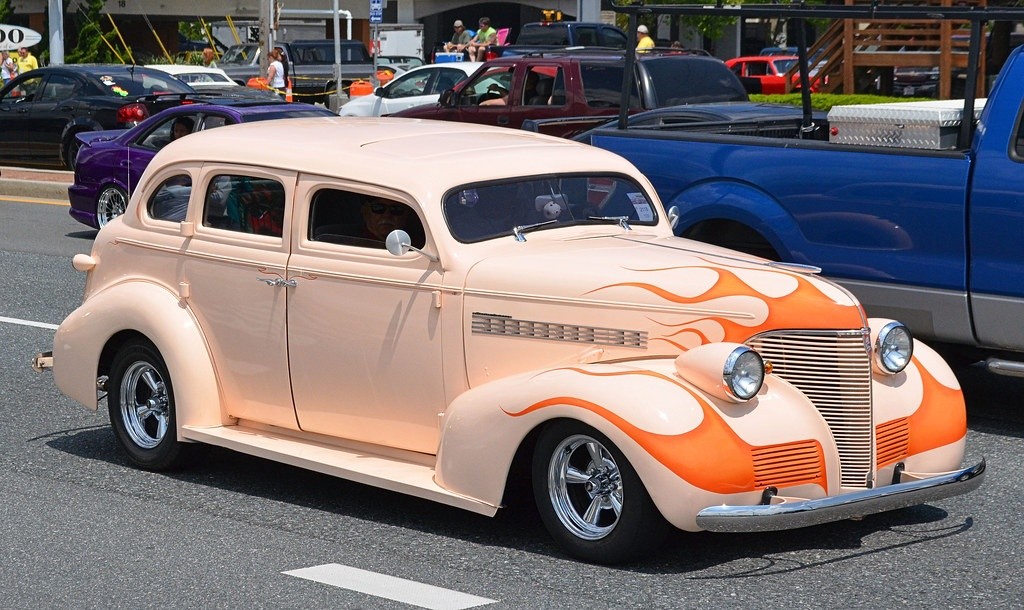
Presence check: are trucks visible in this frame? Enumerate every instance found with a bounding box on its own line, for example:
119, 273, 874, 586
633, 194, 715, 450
371, 23, 426, 61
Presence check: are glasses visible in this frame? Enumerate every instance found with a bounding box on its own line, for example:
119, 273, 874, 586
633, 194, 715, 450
364, 203, 409, 215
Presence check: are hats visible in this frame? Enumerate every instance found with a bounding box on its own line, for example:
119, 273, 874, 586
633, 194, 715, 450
454, 20, 462, 27
637, 25, 648, 33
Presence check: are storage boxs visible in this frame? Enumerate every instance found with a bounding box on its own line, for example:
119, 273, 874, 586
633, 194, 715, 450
434, 52, 465, 63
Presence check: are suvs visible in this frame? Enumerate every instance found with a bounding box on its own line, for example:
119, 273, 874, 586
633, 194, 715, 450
761, 46, 832, 64
514, 21, 628, 48
213, 37, 376, 106
383, 46, 750, 139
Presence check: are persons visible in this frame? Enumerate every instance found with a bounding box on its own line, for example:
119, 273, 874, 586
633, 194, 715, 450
479, 72, 540, 106
265, 47, 289, 101
152, 175, 192, 223
206, 175, 232, 216
0, 47, 39, 98
668, 40, 682, 54
160, 116, 196, 148
334, 197, 408, 249
635, 25, 655, 53
454, 182, 544, 238
195, 48, 218, 83
443, 17, 498, 63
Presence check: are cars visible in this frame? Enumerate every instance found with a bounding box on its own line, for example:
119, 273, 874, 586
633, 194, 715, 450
192, 85, 286, 104
569, 92, 833, 145
64, 102, 342, 234
723, 50, 830, 97
369, 55, 427, 87
0, 62, 241, 172
146, 63, 240, 87
37, 114, 995, 559
337, 57, 505, 121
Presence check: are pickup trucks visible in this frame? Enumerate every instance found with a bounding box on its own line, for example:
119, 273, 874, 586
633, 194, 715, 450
592, 45, 1024, 385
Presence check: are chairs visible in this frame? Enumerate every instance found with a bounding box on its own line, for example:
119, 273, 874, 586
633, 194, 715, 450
496, 27, 512, 46
528, 78, 553, 105
